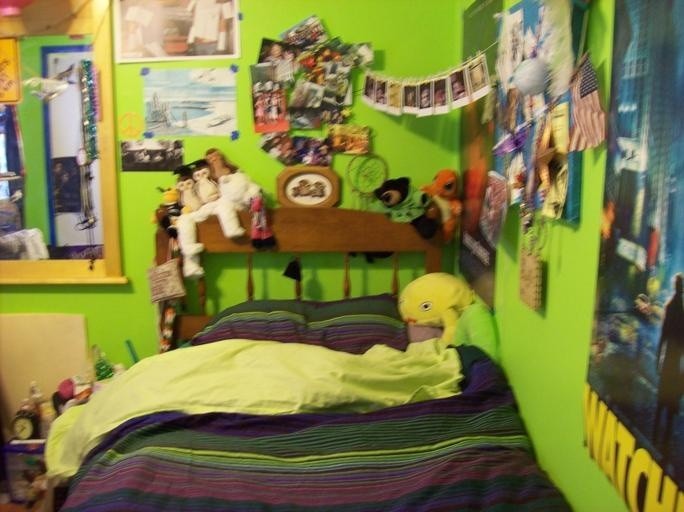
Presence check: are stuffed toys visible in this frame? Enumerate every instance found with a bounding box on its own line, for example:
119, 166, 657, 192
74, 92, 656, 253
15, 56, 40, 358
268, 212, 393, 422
374, 168, 504, 365
150, 146, 276, 279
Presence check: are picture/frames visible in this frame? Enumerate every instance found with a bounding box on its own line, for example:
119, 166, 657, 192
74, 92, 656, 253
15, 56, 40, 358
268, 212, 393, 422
1, 0, 128, 286
111, 0, 241, 64
277, 165, 340, 209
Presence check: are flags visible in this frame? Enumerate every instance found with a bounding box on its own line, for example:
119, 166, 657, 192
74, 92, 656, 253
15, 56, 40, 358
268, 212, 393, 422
568, 60, 606, 152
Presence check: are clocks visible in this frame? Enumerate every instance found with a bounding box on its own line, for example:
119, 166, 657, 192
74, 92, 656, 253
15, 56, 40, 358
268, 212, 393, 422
12, 411, 41, 442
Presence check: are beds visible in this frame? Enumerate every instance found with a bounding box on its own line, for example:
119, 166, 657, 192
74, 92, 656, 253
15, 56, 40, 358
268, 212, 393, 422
61, 205, 575, 512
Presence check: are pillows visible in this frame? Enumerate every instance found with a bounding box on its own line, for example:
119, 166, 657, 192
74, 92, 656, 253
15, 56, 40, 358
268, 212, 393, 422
201, 299, 307, 324
307, 296, 398, 324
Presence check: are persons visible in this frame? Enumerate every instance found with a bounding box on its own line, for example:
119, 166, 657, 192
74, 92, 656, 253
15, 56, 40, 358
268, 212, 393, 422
653, 271, 684, 453
122, 0, 229, 58
